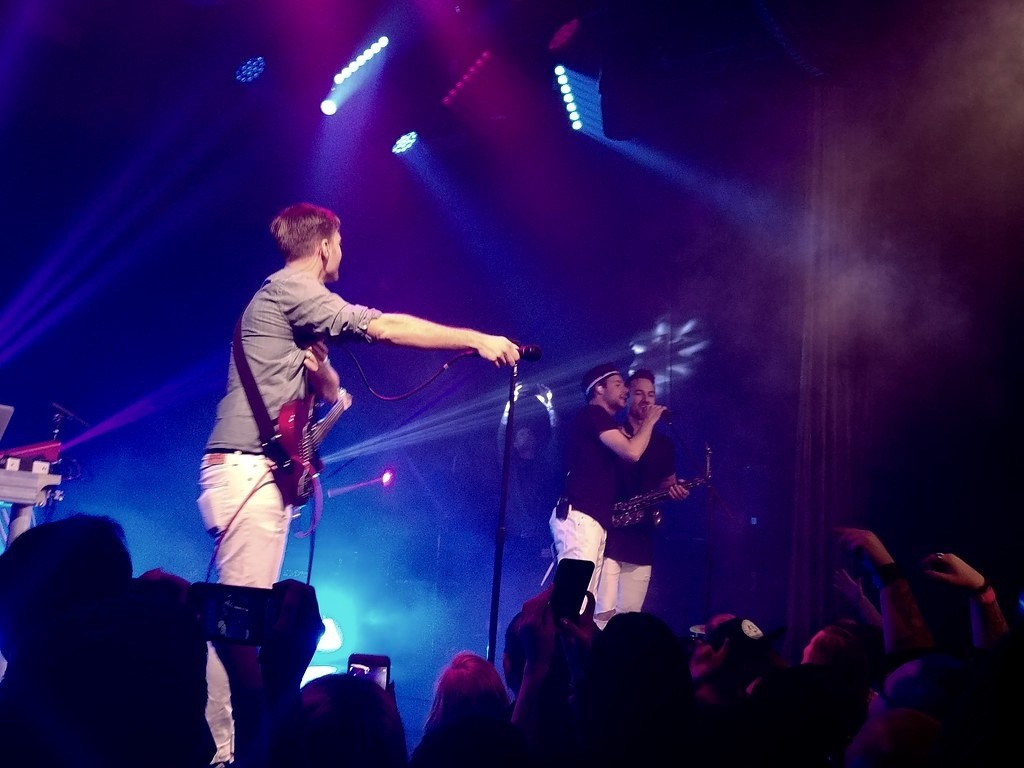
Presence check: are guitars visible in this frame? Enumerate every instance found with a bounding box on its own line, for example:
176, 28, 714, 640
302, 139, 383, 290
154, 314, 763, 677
277, 394, 355, 497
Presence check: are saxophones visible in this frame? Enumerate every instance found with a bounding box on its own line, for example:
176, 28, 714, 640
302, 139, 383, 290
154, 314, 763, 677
611, 441, 714, 529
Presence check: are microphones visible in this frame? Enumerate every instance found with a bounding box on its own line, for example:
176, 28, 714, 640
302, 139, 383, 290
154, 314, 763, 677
470, 340, 543, 363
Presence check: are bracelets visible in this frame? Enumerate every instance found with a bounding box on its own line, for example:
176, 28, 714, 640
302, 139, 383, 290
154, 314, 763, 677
971, 575, 992, 599
870, 561, 908, 590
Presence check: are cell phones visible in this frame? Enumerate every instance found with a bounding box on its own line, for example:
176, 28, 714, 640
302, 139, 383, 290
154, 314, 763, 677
188, 581, 272, 646
345, 653, 390, 692
549, 557, 596, 627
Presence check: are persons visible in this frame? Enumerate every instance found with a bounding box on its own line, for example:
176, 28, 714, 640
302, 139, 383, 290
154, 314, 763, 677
575, 371, 690, 631
194, 202, 522, 768
0, 510, 1024, 768
547, 362, 668, 620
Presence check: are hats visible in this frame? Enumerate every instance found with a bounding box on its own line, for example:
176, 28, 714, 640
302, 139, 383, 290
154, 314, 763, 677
581, 363, 622, 396
710, 618, 789, 668
688, 613, 736, 634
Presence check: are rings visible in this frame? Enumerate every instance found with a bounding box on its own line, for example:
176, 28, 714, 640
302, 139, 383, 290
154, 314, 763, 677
935, 550, 947, 561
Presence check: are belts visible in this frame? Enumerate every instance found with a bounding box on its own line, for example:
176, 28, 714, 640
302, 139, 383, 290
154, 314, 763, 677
203, 447, 264, 456
